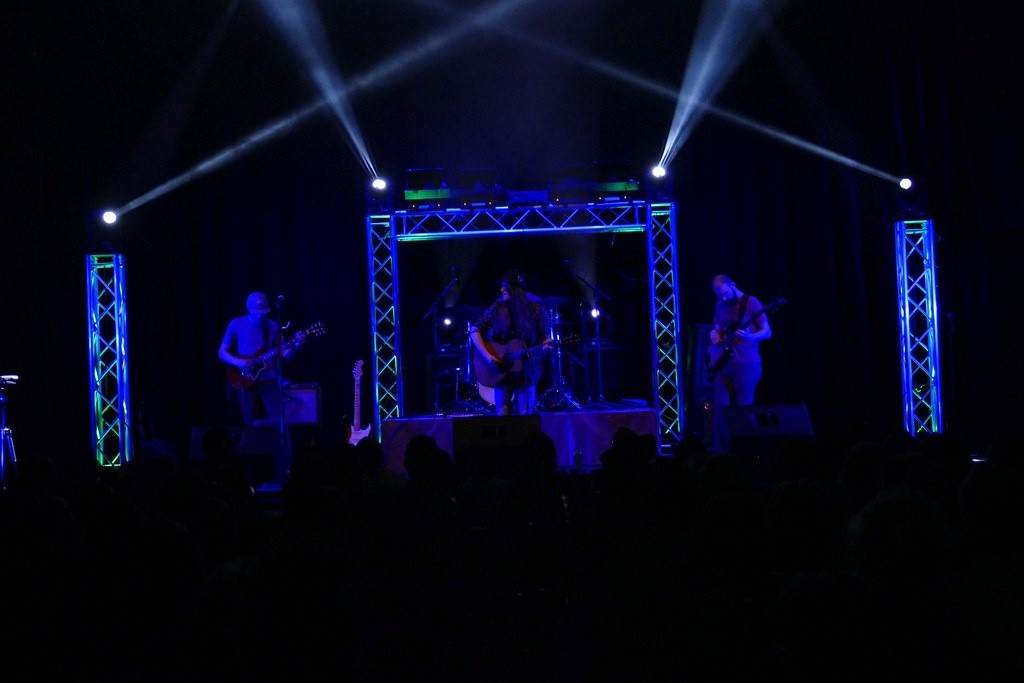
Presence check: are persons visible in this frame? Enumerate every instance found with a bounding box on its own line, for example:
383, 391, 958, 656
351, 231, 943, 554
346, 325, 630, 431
473, 272, 556, 417
711, 275, 771, 449
219, 293, 304, 426
0, 431, 1024, 539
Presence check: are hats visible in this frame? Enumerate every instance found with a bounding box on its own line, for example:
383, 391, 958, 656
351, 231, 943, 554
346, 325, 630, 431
244, 290, 270, 314
499, 271, 529, 292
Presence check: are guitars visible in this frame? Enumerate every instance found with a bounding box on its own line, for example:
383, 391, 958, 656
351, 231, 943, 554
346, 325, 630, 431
705, 297, 786, 369
228, 321, 329, 386
473, 328, 583, 383
344, 358, 372, 446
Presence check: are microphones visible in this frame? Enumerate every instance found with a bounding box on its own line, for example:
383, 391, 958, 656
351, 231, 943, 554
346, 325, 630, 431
275, 295, 287, 311
